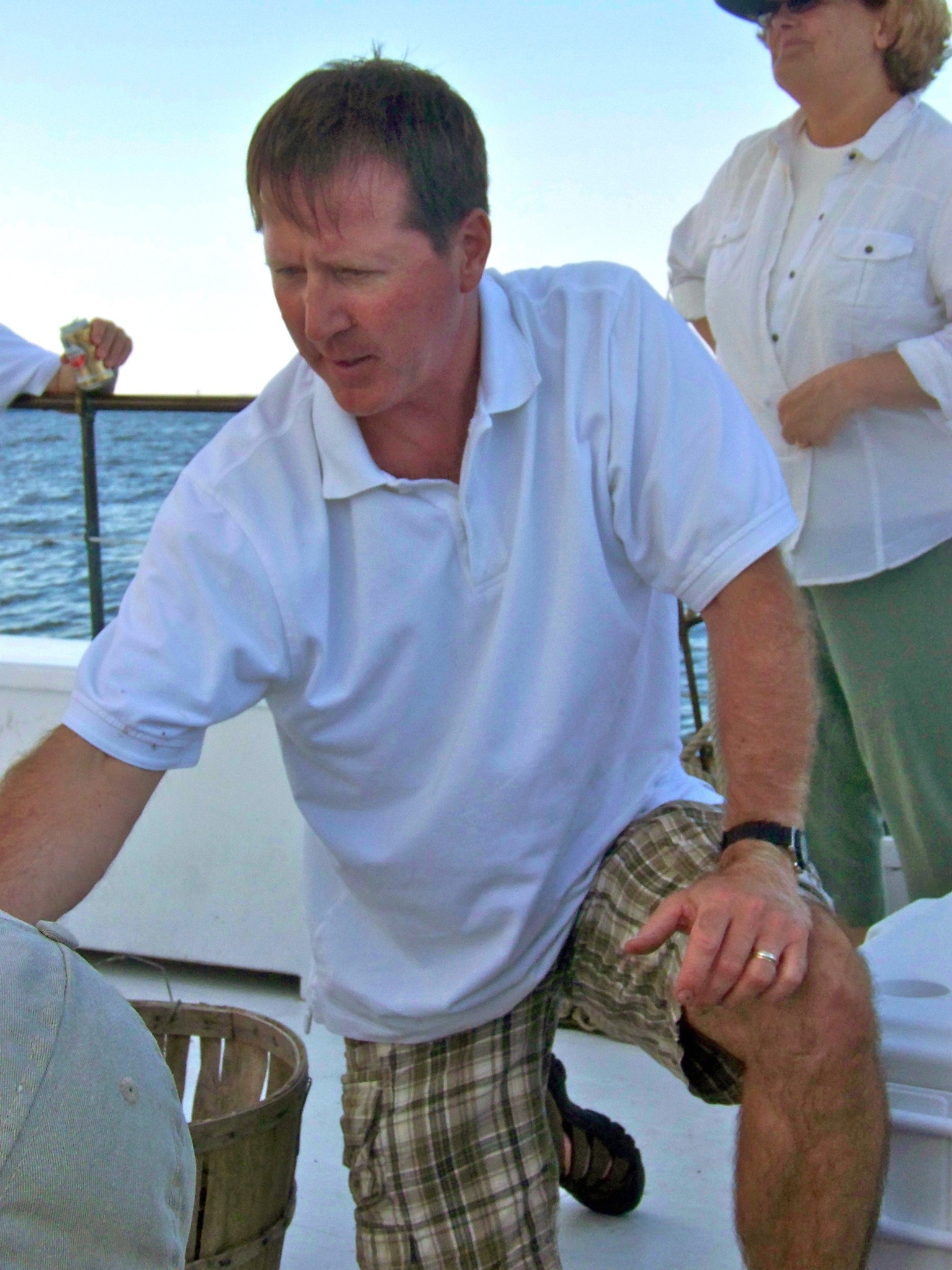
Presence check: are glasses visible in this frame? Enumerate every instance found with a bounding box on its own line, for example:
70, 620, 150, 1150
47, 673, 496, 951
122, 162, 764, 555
756, 0, 822, 28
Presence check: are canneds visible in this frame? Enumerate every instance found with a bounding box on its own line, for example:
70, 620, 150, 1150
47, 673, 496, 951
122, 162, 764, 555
60, 318, 117, 393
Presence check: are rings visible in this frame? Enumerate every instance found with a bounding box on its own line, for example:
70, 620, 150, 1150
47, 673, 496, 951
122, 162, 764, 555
753, 949, 780, 968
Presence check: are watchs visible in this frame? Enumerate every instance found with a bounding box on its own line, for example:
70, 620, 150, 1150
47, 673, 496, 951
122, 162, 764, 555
715, 814, 809, 874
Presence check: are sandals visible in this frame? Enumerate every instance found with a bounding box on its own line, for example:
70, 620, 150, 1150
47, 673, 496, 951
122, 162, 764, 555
547, 1054, 646, 1215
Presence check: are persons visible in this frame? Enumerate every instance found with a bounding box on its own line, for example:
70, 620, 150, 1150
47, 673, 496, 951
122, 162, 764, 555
669, 0, 950, 950
0, 52, 890, 1269
0, 316, 133, 411
